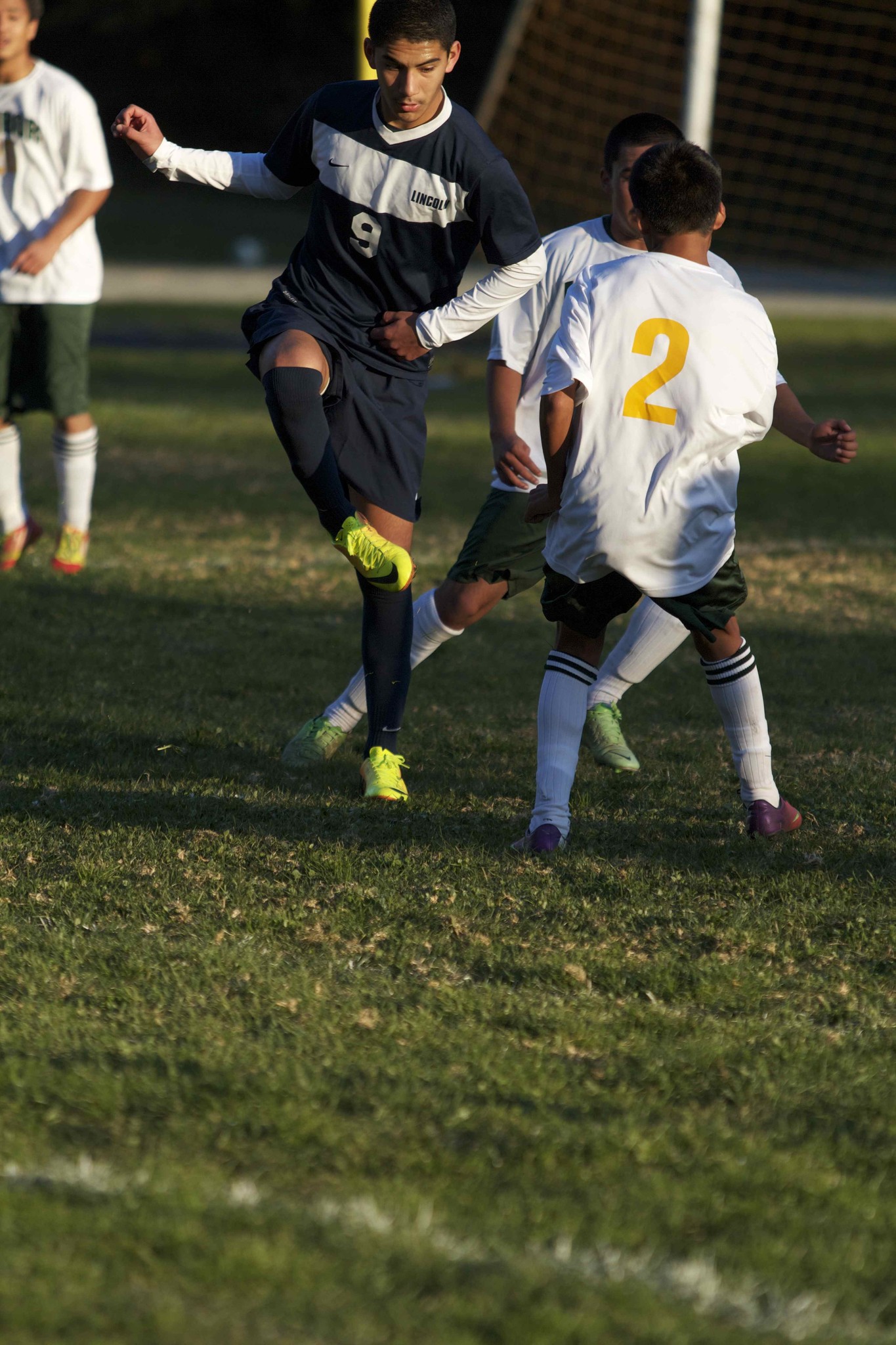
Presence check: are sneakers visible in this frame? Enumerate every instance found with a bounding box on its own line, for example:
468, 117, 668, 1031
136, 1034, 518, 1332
581, 699, 641, 773
744, 797, 803, 837
281, 711, 349, 765
0, 515, 43, 572
330, 511, 416, 592
511, 824, 568, 857
362, 746, 410, 802
51, 525, 90, 575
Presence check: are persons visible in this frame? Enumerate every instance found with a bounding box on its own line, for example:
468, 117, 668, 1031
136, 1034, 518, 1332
275, 110, 861, 770
502, 139, 804, 855
0, 0, 112, 575
112, 0, 552, 801
